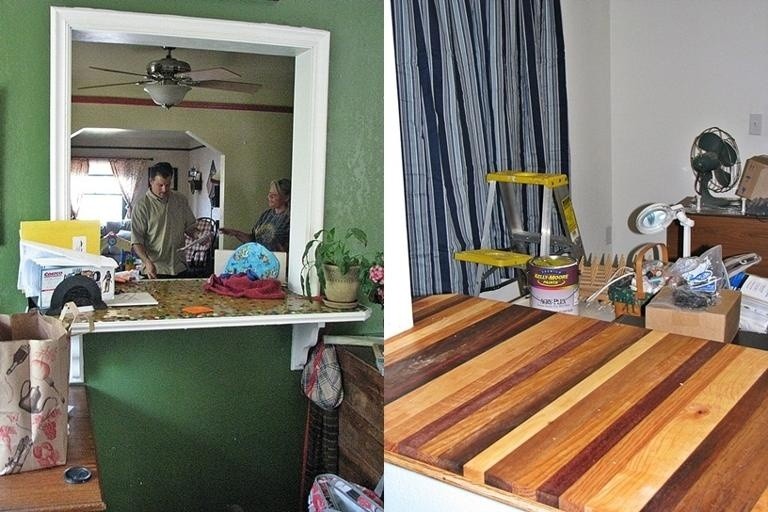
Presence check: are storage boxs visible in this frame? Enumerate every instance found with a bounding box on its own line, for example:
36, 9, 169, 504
736, 154, 768, 202
645, 282, 741, 343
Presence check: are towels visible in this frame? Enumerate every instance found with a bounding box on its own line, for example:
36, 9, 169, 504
205, 274, 286, 299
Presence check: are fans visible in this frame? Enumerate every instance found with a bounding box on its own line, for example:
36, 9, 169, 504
690, 126, 743, 207
78, 47, 262, 94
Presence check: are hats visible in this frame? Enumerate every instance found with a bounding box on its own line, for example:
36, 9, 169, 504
45, 276, 108, 316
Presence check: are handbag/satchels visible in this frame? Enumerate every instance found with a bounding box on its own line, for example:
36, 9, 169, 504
0, 310, 69, 475
308, 473, 384, 512
301, 343, 344, 410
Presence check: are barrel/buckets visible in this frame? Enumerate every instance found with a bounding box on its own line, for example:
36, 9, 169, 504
525, 255, 580, 315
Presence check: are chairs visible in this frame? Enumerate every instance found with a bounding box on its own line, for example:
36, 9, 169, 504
184, 217, 218, 277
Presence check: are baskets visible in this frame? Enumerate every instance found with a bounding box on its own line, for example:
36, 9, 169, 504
614, 242, 668, 316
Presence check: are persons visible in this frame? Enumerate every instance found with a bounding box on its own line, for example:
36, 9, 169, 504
218, 177, 292, 251
101, 271, 114, 293
130, 161, 211, 279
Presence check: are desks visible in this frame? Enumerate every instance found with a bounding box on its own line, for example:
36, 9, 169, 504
666, 197, 768, 280
0, 385, 107, 512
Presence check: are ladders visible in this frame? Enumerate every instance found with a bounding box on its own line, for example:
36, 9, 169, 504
455, 170, 587, 297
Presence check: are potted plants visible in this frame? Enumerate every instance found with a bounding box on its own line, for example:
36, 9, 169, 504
299, 228, 369, 309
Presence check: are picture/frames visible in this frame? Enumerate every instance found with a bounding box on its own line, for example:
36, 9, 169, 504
148, 167, 178, 191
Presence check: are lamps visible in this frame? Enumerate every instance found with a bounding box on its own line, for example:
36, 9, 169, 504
143, 79, 192, 110
188, 165, 202, 193
636, 203, 695, 259
